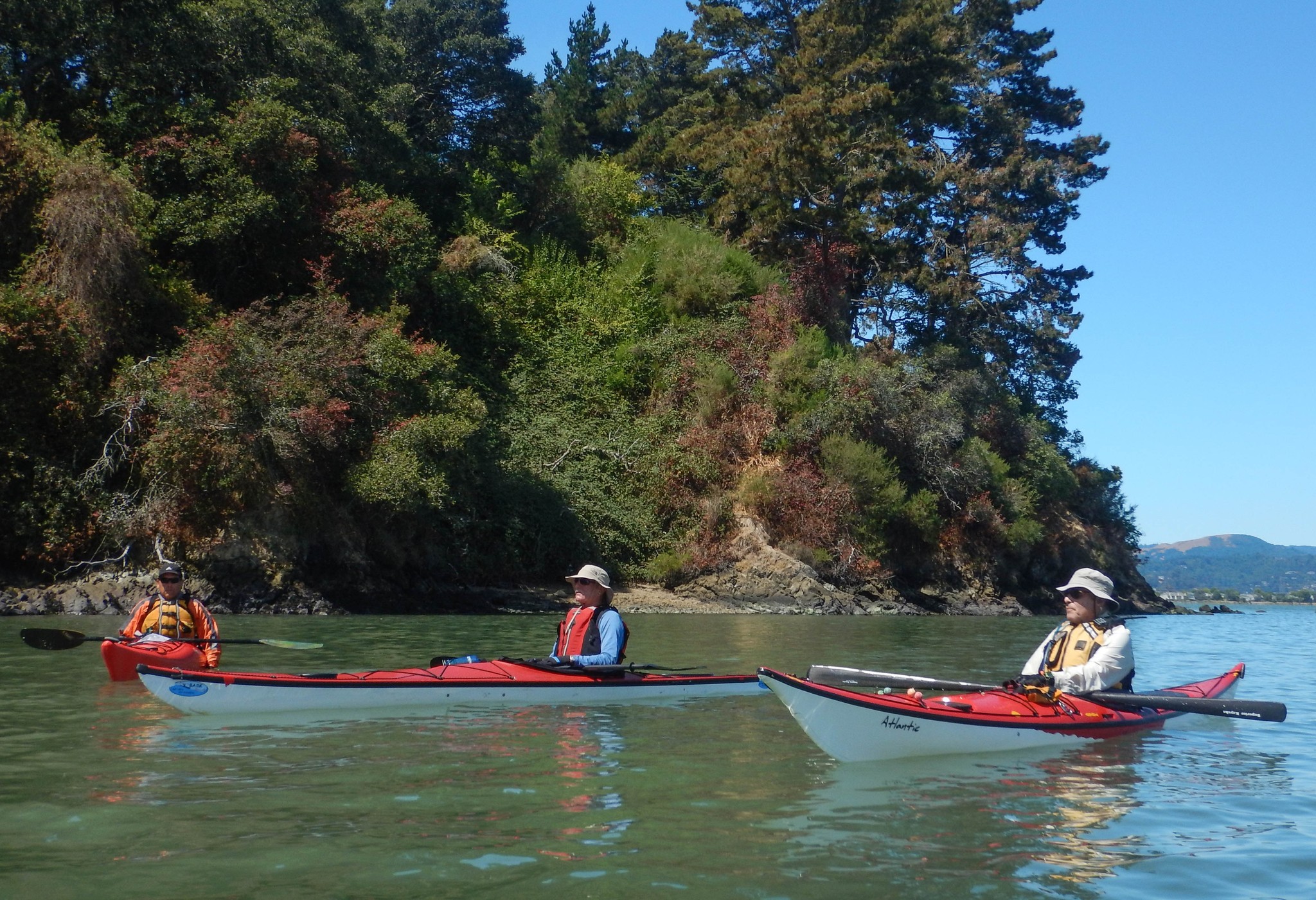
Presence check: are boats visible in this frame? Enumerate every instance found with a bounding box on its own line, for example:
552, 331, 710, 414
756, 662, 1249, 767
100, 635, 208, 684
135, 661, 763, 718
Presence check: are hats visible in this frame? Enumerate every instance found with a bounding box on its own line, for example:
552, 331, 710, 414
565, 564, 611, 589
1055, 568, 1119, 612
158, 563, 183, 579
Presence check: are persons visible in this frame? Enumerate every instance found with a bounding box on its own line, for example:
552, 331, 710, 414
1016, 568, 1135, 695
118, 563, 222, 668
528, 565, 630, 668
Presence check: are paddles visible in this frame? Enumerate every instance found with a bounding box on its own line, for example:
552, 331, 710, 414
805, 665, 1288, 722
19, 627, 325, 650
580, 662, 708, 672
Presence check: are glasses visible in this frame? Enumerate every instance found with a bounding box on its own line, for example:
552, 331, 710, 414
158, 577, 180, 583
575, 578, 596, 585
1064, 588, 1090, 599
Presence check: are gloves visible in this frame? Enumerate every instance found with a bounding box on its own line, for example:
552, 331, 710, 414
1016, 674, 1055, 687
528, 655, 572, 667
